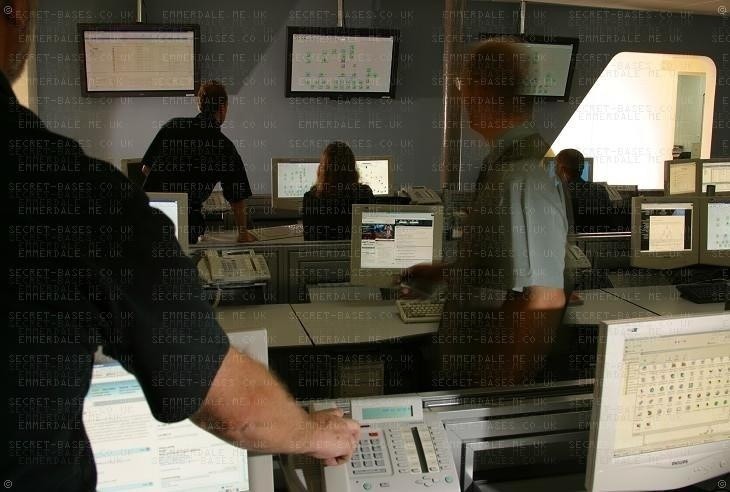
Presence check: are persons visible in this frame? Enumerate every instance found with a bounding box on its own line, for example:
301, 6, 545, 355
140, 79, 261, 245
1, 2, 364, 492
393, 37, 572, 388
552, 149, 590, 187
300, 141, 375, 242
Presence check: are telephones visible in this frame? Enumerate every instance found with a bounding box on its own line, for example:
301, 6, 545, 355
597, 182, 623, 208
197, 249, 271, 289
278, 397, 462, 491
203, 194, 228, 213
569, 245, 591, 274
396, 184, 442, 205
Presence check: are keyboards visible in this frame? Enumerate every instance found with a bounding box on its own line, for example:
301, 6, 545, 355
247, 224, 304, 242
395, 298, 445, 323
675, 280, 730, 304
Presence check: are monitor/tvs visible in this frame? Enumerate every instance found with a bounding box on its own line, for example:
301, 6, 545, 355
82, 326, 275, 492
698, 195, 730, 267
355, 155, 395, 198
663, 159, 699, 196
543, 157, 593, 186
121, 158, 146, 188
77, 22, 202, 98
630, 196, 700, 271
479, 32, 580, 104
350, 203, 444, 290
284, 25, 401, 100
584, 311, 730, 492
146, 192, 189, 257
700, 158, 730, 196
270, 157, 321, 210
